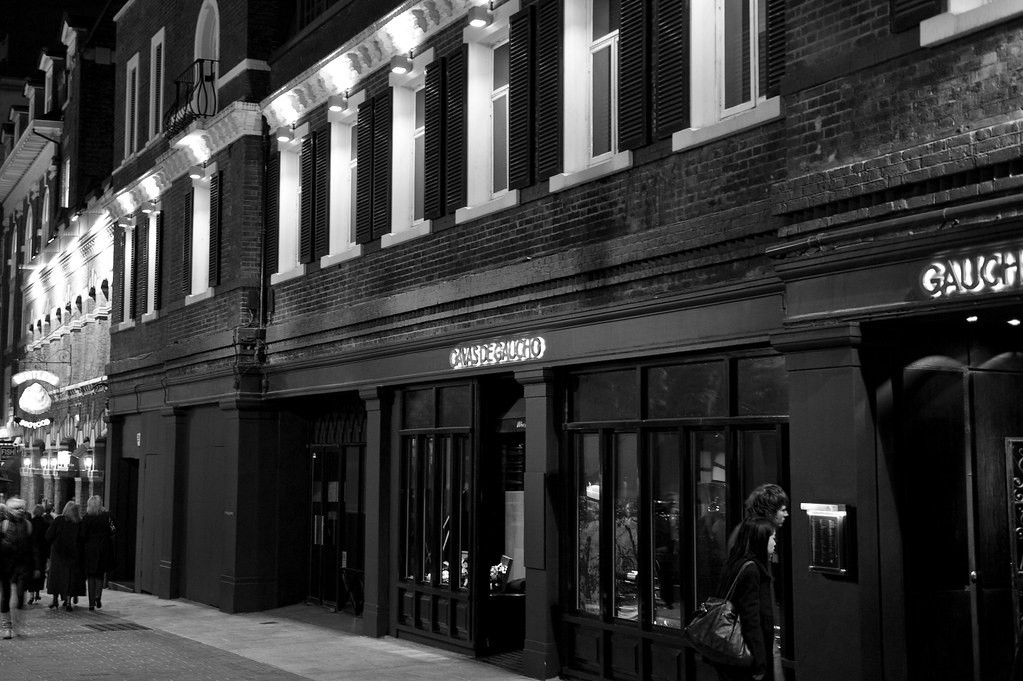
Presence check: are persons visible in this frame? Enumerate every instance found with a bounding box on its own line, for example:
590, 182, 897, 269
653, 491, 682, 610
0, 495, 123, 639
701, 481, 789, 681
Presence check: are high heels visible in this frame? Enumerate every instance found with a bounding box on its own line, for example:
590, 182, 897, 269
27, 598, 34, 605
48, 602, 59, 610
34, 596, 41, 601
66, 603, 72, 612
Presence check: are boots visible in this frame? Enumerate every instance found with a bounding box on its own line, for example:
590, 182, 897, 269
0, 611, 12, 639
13, 607, 28, 637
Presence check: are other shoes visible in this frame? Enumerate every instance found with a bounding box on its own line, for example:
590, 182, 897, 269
62, 601, 66, 607
73, 596, 78, 604
96, 598, 102, 608
88, 606, 94, 611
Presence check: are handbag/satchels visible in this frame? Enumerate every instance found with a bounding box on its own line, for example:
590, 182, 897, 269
108, 511, 116, 538
680, 562, 759, 669
